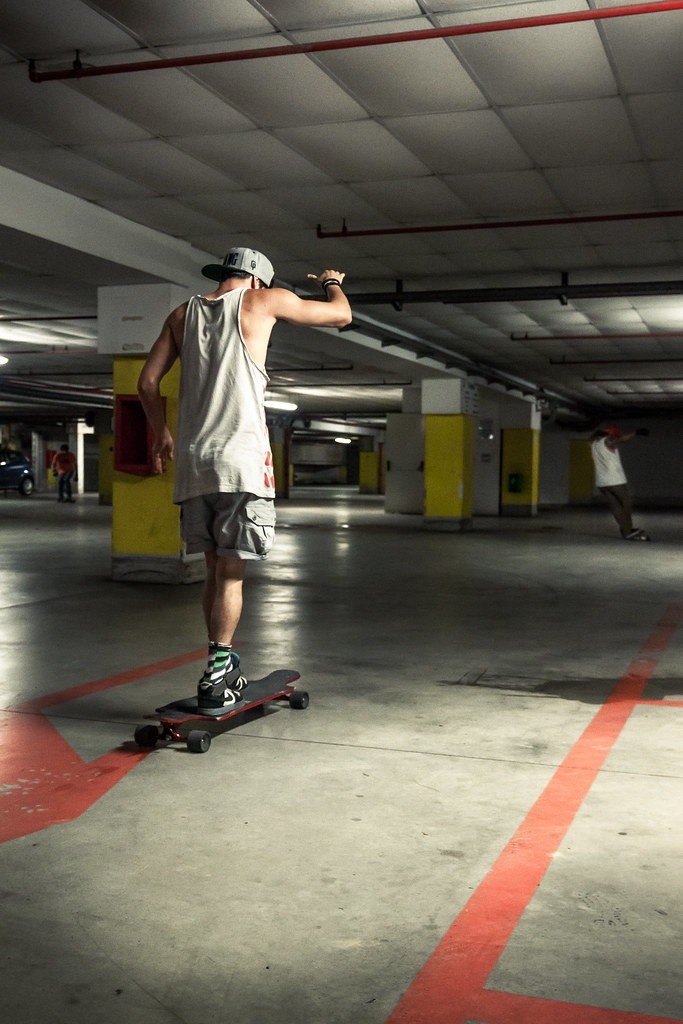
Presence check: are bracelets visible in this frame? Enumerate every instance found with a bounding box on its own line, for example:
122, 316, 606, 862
322, 279, 340, 295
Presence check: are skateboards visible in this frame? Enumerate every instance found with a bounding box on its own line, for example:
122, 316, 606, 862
626, 528, 650, 542
58, 498, 75, 503
134, 669, 309, 753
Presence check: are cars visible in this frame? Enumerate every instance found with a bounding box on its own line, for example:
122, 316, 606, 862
0, 449, 34, 497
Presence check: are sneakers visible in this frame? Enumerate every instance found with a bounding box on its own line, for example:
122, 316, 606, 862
196, 677, 244, 715
199, 651, 249, 691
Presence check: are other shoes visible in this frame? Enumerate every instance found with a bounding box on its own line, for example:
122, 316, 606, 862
56, 497, 64, 502
625, 528, 641, 539
65, 497, 71, 502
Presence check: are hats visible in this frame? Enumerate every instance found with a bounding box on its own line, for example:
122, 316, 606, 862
201, 248, 274, 287
604, 426, 622, 437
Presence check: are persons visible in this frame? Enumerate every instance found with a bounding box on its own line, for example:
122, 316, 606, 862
137, 247, 353, 716
590, 425, 650, 540
50, 444, 78, 501
